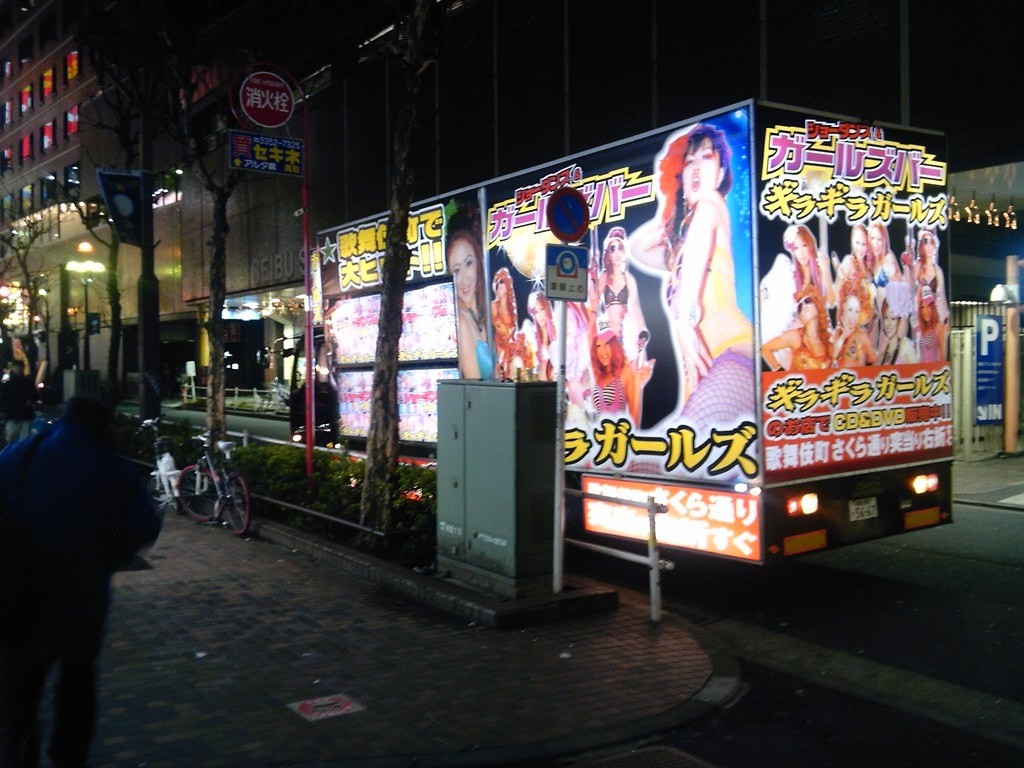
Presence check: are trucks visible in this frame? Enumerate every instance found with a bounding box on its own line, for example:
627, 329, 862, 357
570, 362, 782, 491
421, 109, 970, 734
256, 98, 956, 567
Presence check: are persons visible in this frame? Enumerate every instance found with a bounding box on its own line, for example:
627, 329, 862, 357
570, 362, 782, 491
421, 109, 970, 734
291, 366, 341, 448
579, 325, 656, 427
761, 284, 835, 372
0, 397, 163, 768
0, 361, 39, 444
492, 268, 558, 382
784, 225, 950, 365
627, 125, 757, 482
445, 216, 494, 382
589, 228, 650, 363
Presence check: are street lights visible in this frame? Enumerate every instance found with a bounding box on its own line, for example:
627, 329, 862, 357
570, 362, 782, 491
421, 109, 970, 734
66, 261, 106, 370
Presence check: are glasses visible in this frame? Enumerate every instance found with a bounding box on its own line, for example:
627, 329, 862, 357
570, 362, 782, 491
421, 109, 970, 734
797, 296, 813, 312
496, 279, 504, 291
922, 238, 935, 244
608, 243, 623, 253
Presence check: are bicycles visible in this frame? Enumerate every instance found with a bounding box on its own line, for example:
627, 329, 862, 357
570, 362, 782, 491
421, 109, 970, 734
176, 427, 251, 536
133, 413, 184, 517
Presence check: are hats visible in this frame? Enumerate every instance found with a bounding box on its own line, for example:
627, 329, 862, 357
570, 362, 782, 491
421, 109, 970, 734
918, 226, 940, 245
592, 317, 623, 343
603, 227, 626, 248
919, 287, 936, 306
